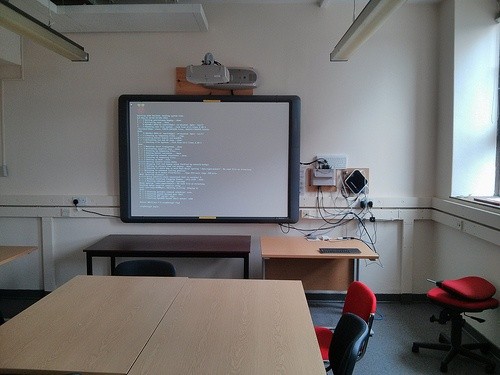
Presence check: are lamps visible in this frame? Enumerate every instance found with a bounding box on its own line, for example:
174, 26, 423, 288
330, 0, 408, 61
0, 0, 89, 62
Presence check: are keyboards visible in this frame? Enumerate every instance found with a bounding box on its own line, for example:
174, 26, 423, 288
319, 248, 360, 253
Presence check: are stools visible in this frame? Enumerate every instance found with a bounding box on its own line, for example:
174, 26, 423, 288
412, 276, 500, 375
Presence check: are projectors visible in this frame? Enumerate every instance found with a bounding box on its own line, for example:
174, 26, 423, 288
186, 52, 230, 83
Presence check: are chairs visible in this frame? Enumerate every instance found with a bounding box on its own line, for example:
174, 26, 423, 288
112, 260, 175, 277
315, 279, 377, 375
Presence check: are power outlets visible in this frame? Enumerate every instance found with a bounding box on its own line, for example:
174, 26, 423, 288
73, 196, 87, 203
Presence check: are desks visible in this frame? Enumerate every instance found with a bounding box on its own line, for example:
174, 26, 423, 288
260, 236, 382, 321
82, 234, 252, 279
0, 275, 188, 375
0, 246, 35, 265
127, 278, 328, 375
